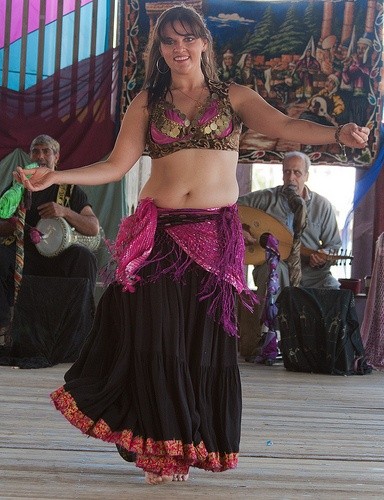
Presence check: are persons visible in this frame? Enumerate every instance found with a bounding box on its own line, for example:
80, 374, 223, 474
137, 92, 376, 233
0, 134, 99, 335
236, 151, 342, 364
10, 5, 370, 484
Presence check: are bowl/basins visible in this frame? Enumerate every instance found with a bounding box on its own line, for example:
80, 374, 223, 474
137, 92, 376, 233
338, 279, 361, 294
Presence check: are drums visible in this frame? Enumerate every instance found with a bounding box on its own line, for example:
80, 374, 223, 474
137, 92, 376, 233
35, 215, 101, 257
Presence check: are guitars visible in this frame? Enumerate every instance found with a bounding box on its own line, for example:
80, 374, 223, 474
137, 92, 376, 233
237, 204, 354, 265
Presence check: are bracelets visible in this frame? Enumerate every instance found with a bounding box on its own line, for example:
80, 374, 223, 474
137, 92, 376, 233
335, 125, 344, 147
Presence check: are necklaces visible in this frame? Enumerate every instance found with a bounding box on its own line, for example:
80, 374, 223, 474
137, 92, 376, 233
172, 83, 205, 109
